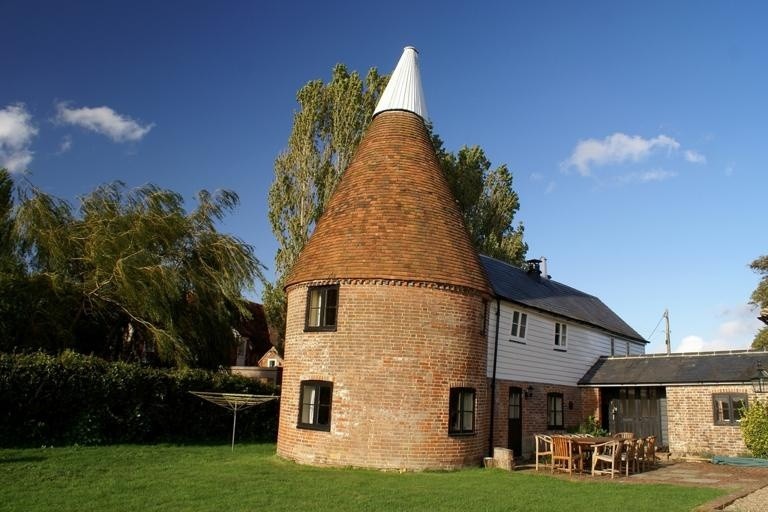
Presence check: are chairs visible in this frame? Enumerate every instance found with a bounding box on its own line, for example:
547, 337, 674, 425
534, 432, 658, 479
